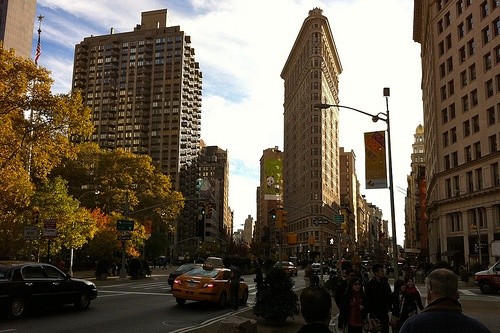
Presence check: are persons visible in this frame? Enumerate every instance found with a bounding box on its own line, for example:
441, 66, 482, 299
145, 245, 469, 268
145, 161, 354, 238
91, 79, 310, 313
226, 262, 242, 311
399, 268, 491, 333
299, 261, 424, 333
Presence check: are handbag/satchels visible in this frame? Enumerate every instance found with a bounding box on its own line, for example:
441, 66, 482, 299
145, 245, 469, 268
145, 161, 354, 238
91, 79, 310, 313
363, 317, 381, 331
390, 315, 401, 327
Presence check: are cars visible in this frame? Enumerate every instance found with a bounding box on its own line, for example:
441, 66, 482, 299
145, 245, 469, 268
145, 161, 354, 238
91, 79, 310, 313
0, 260, 98, 320
273, 261, 298, 275
168, 257, 249, 309
475, 260, 500, 294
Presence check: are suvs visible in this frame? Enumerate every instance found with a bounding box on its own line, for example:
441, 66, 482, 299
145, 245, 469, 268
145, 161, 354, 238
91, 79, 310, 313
311, 262, 331, 274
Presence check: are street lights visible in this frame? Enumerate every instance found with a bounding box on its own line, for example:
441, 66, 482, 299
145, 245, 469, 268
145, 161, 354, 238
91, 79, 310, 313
69, 190, 101, 278
313, 87, 399, 282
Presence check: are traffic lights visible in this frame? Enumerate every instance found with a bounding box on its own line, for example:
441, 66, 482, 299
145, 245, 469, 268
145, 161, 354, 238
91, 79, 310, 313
345, 247, 350, 253
201, 208, 206, 216
269, 213, 277, 221
326, 237, 335, 246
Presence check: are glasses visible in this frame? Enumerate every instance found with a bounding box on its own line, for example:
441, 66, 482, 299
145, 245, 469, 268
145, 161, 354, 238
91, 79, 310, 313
346, 274, 357, 277
353, 283, 361, 286
